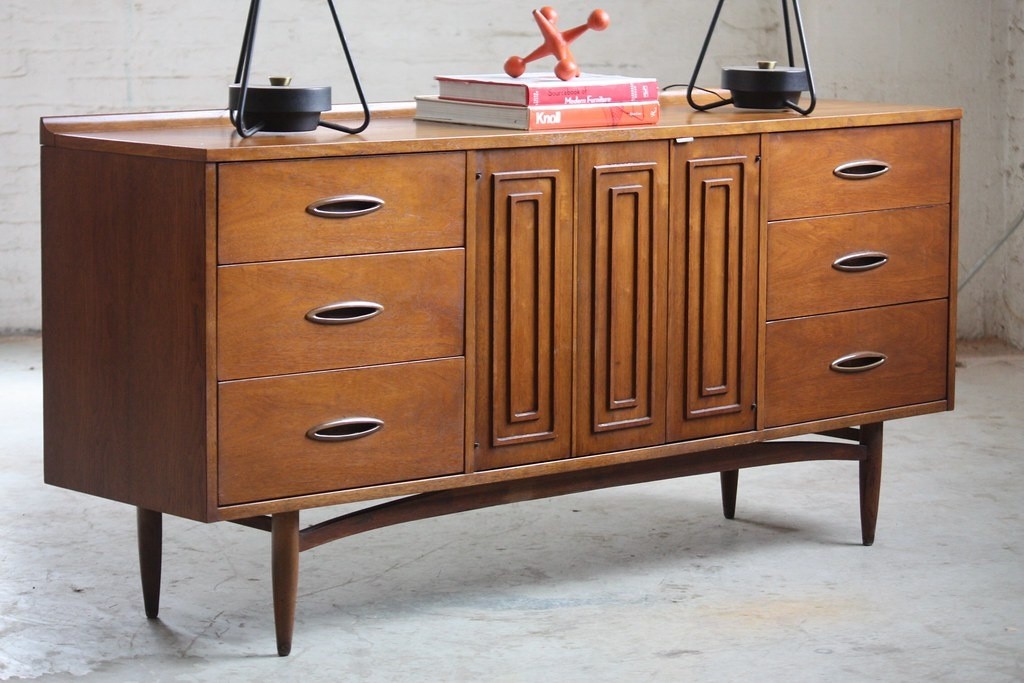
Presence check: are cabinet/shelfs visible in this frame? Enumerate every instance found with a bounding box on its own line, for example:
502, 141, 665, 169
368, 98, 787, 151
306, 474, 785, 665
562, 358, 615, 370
40, 87, 964, 657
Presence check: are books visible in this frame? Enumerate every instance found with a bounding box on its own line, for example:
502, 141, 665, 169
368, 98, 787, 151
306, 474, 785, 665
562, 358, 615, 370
435, 72, 658, 107
413, 95, 659, 131
